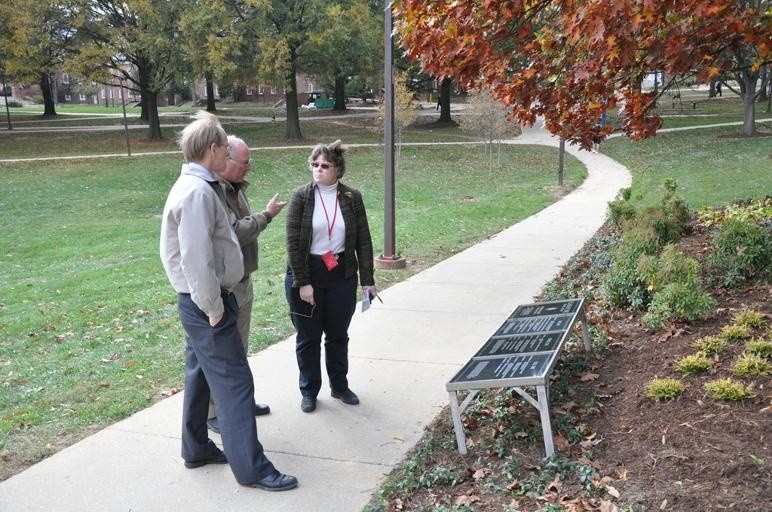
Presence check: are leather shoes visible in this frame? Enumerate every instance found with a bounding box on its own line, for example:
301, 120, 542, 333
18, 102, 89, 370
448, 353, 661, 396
184, 449, 227, 469
250, 469, 298, 491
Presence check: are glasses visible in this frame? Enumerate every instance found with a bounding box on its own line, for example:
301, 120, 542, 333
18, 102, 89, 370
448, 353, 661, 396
311, 163, 334, 169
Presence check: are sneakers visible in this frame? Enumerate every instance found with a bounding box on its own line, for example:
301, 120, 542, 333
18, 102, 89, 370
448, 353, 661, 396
301, 396, 316, 412
331, 388, 359, 405
256, 404, 270, 415
208, 418, 220, 433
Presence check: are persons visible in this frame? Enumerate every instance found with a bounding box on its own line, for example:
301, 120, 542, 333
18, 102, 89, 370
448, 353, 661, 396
435, 94, 442, 112
590, 103, 606, 156
209, 135, 287, 433
160, 109, 299, 491
714, 79, 723, 98
283, 140, 377, 411
